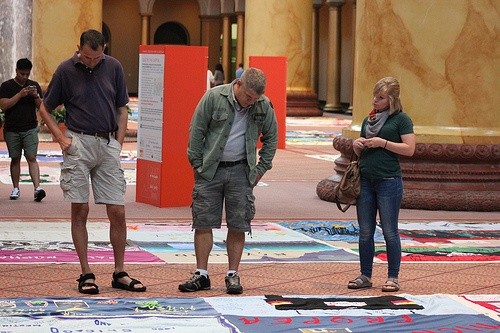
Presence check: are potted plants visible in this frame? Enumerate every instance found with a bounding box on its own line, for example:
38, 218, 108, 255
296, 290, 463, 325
0, 109, 5, 141
39, 106, 65, 143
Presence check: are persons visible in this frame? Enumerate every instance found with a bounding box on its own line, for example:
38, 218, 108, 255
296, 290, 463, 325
207, 63, 244, 90
347, 76, 415, 292
178, 66, 278, 294
0, 57, 46, 201
40, 30, 147, 294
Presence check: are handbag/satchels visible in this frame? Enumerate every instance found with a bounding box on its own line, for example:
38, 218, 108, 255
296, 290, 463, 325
336, 144, 365, 212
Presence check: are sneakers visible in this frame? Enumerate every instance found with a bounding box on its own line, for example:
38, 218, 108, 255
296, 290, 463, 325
10, 188, 20, 199
225, 273, 243, 294
34, 187, 46, 202
179, 271, 210, 292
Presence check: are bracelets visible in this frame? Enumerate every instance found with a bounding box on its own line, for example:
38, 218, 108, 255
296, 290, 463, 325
33, 93, 40, 99
383, 140, 388, 149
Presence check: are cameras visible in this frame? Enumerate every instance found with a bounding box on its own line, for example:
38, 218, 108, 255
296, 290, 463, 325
28, 86, 35, 94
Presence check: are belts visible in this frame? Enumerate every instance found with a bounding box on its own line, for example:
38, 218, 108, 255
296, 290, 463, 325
68, 127, 109, 139
218, 158, 248, 168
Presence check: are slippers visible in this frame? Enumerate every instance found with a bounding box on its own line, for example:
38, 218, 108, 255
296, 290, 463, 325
382, 278, 399, 291
348, 275, 373, 288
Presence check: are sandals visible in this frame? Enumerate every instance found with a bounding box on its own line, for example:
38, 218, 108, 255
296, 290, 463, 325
76, 273, 99, 294
112, 271, 146, 292
